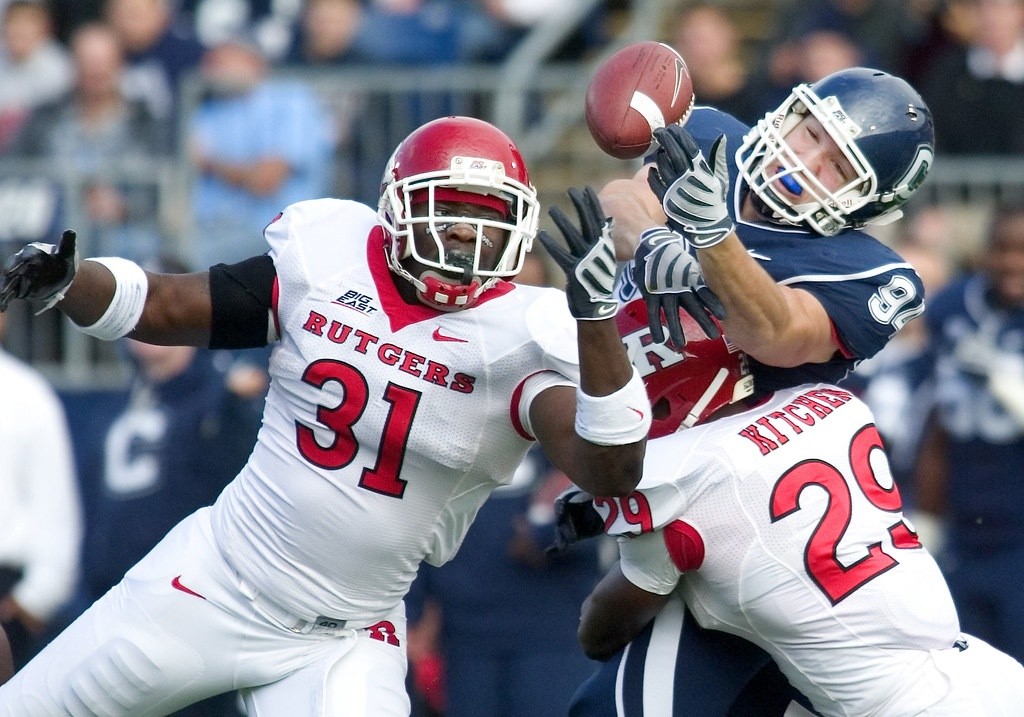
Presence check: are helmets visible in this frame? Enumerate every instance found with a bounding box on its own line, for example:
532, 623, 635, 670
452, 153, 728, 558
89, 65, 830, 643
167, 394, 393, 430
378, 115, 540, 311
733, 67, 934, 236
611, 297, 756, 439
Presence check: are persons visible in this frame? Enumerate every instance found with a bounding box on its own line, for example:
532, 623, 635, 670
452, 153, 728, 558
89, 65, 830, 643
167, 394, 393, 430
0, 0, 1023, 717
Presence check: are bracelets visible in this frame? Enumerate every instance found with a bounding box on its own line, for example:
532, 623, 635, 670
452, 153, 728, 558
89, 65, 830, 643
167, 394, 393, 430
574, 366, 653, 445
69, 249, 147, 339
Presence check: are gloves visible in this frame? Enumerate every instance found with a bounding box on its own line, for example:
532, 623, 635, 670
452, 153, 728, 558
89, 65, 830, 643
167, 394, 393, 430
1, 230, 79, 316
648, 124, 734, 250
539, 186, 618, 320
634, 227, 727, 347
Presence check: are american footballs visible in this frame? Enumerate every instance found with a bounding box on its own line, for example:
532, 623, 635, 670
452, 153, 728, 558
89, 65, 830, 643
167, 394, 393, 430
584, 42, 691, 161
